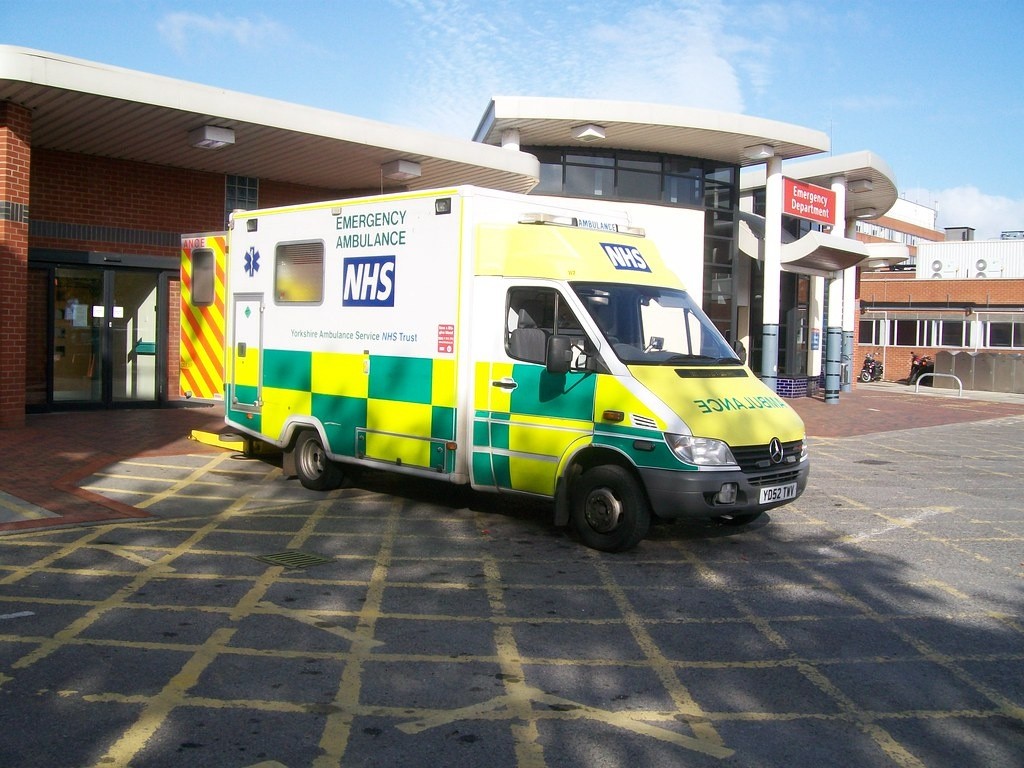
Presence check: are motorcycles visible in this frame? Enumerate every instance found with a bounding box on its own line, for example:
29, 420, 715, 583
860, 352, 883, 383
908, 351, 933, 386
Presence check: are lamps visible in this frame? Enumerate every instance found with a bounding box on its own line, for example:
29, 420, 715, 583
854, 207, 876, 219
847, 180, 872, 194
744, 143, 774, 160
187, 126, 236, 151
570, 124, 605, 143
382, 160, 422, 182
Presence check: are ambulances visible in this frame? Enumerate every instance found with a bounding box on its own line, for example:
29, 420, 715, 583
178, 184, 812, 554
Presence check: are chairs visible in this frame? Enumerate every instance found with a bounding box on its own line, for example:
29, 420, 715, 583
585, 305, 619, 352
510, 300, 549, 363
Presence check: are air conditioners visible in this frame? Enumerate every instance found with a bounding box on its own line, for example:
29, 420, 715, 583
928, 255, 959, 272
928, 272, 957, 279
971, 272, 1002, 278
971, 256, 1002, 272
